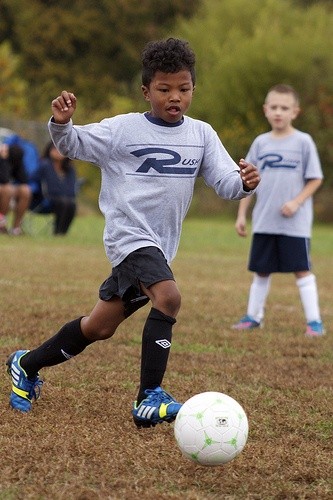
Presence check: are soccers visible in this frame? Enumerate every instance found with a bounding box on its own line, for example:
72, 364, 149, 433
173, 391, 249, 466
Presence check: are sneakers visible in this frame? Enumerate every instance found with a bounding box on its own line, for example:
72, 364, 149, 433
5, 350, 44, 414
133, 386, 183, 428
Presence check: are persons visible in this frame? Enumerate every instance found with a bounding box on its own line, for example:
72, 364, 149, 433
0, 141, 32, 236
7, 39, 261, 428
28, 140, 78, 235
229, 84, 325, 340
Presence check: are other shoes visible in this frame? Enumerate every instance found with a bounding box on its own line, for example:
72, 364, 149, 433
232, 316, 264, 330
304, 320, 324, 337
